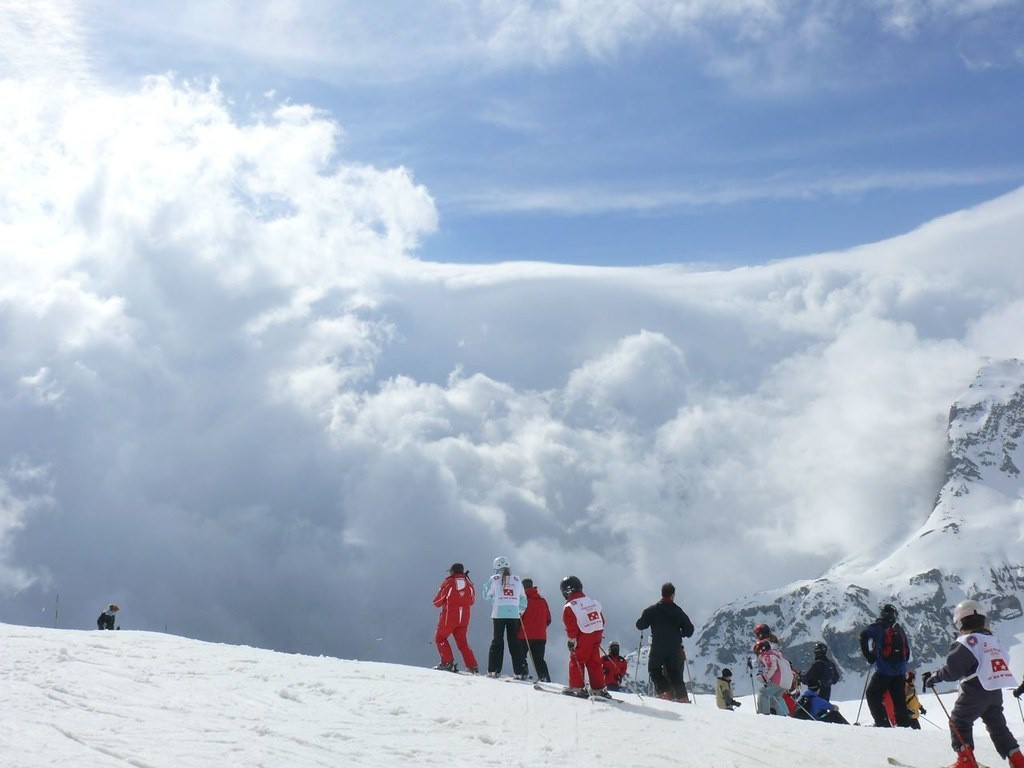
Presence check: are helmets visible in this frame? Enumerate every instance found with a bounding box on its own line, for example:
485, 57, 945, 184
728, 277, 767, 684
492, 557, 510, 570
608, 640, 619, 652
906, 671, 916, 685
753, 640, 771, 655
814, 643, 827, 656
753, 624, 772, 638
560, 576, 583, 599
953, 600, 986, 631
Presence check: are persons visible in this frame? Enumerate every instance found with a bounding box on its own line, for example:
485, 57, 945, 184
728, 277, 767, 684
924, 600, 1024, 768
481, 556, 928, 731
433, 563, 480, 676
96, 604, 120, 631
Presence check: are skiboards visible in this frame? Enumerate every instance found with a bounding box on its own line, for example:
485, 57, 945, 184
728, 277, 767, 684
483, 669, 537, 685
431, 664, 477, 678
534, 682, 622, 706
886, 755, 990, 768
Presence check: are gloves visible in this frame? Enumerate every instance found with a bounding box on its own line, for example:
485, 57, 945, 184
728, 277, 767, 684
926, 669, 942, 688
567, 638, 577, 651
732, 700, 741, 707
865, 652, 876, 665
919, 706, 927, 716
1013, 687, 1022, 698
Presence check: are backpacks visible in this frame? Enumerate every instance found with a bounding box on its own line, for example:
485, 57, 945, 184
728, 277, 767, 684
793, 695, 823, 720
875, 618, 910, 663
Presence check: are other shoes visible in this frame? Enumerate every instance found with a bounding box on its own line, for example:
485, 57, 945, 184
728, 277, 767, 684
944, 746, 978, 768
486, 671, 499, 678
512, 673, 527, 681
463, 664, 480, 673
1009, 749, 1024, 768
433, 661, 458, 672
562, 687, 582, 694
587, 688, 605, 696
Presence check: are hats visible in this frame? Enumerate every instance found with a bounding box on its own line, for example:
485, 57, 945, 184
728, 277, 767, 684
447, 563, 464, 573
807, 678, 821, 690
722, 668, 732, 676
